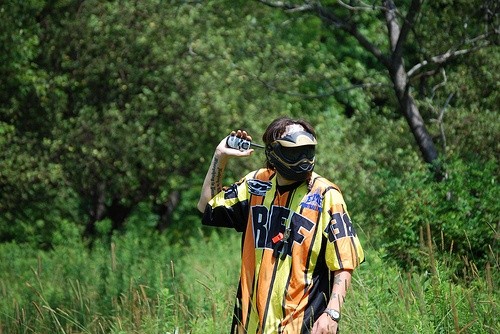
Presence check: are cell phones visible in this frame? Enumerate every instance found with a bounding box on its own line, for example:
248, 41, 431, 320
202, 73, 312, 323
227, 136, 265, 152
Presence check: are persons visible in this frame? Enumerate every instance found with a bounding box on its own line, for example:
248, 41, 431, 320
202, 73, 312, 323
196, 117, 365, 334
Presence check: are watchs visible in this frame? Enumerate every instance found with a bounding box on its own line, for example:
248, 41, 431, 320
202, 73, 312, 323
323, 307, 341, 323
